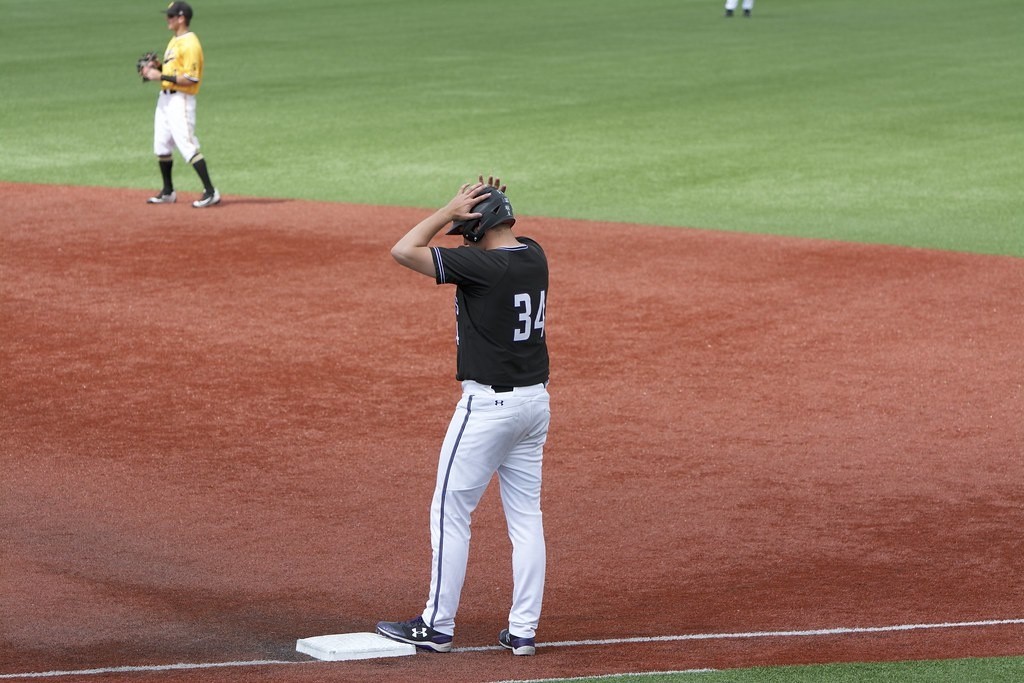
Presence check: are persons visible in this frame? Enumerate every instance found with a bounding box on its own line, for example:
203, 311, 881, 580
137, 0, 220, 209
723, 0, 753, 18
376, 173, 551, 656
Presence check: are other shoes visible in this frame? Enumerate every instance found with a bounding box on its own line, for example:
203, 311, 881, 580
724, 10, 733, 16
744, 9, 750, 16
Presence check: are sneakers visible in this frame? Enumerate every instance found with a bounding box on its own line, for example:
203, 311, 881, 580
376, 615, 454, 652
498, 628, 536, 655
147, 189, 176, 203
192, 188, 220, 207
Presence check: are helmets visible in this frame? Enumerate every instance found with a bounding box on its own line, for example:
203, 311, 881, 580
445, 186, 516, 243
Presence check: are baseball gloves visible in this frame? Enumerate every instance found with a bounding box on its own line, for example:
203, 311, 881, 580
135, 51, 162, 84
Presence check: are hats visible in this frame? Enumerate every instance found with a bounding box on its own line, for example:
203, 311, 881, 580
160, 1, 193, 17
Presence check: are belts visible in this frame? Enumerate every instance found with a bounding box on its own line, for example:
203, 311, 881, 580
164, 89, 176, 94
491, 383, 545, 393
